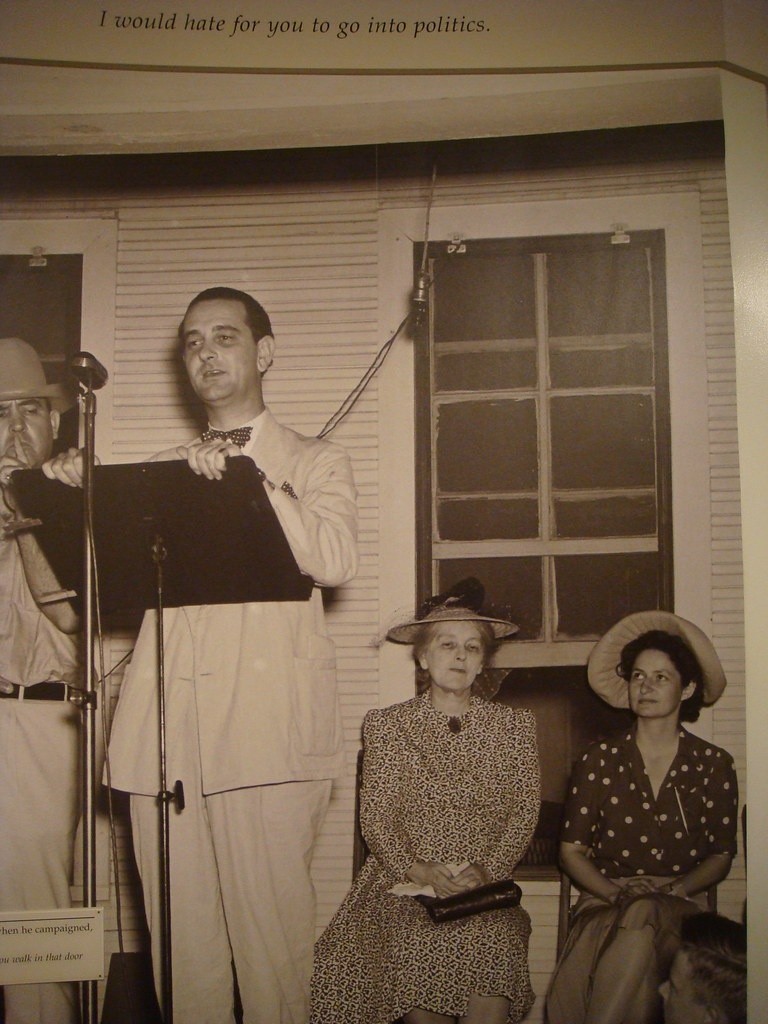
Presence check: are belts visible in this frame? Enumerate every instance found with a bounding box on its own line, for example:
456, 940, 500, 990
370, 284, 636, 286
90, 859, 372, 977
0, 682, 78, 700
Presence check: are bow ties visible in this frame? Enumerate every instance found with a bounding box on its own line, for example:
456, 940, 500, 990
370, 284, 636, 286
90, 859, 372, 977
202, 426, 253, 449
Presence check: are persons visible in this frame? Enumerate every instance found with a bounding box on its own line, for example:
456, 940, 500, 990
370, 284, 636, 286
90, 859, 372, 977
309, 577, 543, 1024
657, 910, 747, 1024
41, 287, 357, 1024
545, 610, 739, 1024
0, 337, 78, 1024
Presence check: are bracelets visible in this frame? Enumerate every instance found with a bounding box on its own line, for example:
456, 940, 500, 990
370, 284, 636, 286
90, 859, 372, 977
668, 882, 674, 892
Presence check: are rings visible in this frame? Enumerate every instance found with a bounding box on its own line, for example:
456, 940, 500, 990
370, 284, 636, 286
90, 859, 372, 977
218, 448, 229, 458
476, 878, 481, 886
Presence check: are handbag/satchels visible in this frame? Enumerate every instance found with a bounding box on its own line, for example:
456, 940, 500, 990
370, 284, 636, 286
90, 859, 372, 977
413, 879, 522, 923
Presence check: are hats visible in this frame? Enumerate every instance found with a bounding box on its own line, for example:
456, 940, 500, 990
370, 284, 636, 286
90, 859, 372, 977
0, 336, 76, 414
370, 577, 520, 647
587, 610, 726, 709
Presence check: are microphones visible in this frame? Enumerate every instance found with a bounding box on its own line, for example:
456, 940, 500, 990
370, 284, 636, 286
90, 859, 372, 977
71, 352, 108, 390
